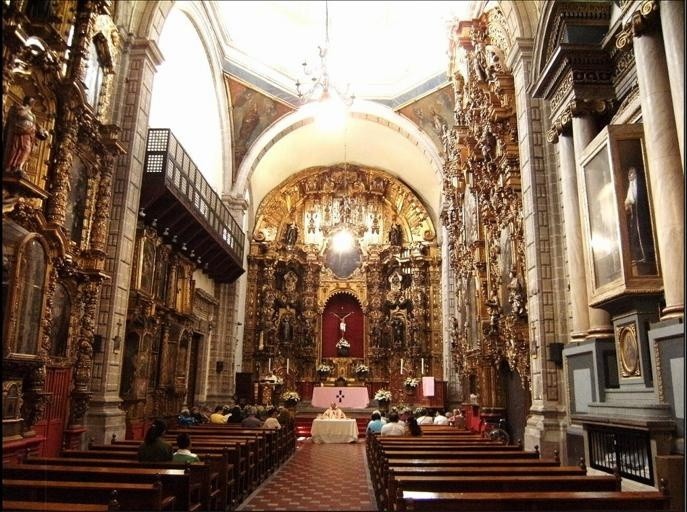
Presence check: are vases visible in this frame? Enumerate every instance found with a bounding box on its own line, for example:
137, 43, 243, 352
359, 378, 367, 383
407, 388, 417, 395
321, 375, 328, 382
286, 404, 297, 412
379, 401, 389, 412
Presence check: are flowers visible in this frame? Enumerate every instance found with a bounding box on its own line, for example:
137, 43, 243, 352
317, 363, 331, 376
401, 378, 422, 388
356, 365, 371, 376
282, 392, 302, 406
374, 390, 392, 402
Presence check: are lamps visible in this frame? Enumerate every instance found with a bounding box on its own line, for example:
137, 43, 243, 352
295, 0, 357, 110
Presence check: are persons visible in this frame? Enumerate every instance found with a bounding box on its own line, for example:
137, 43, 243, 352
506, 269, 527, 315
330, 311, 353, 340
322, 401, 346, 419
369, 222, 420, 347
172, 433, 200, 462
263, 220, 315, 349
9, 94, 46, 178
137, 419, 171, 461
484, 288, 504, 334
365, 406, 463, 436
175, 403, 289, 430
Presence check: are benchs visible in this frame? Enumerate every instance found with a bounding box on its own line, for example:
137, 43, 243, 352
0, 412, 295, 512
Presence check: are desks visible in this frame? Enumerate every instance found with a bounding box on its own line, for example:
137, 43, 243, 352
310, 418, 360, 444
310, 386, 370, 409
